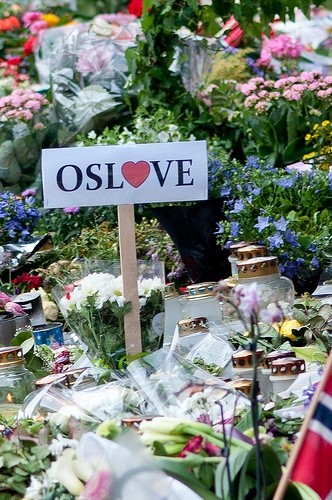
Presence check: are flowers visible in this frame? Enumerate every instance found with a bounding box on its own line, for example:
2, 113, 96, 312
0, 0, 332, 500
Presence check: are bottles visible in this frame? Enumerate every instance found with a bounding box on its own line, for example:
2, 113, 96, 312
0, 346, 37, 409
229, 256, 294, 323
